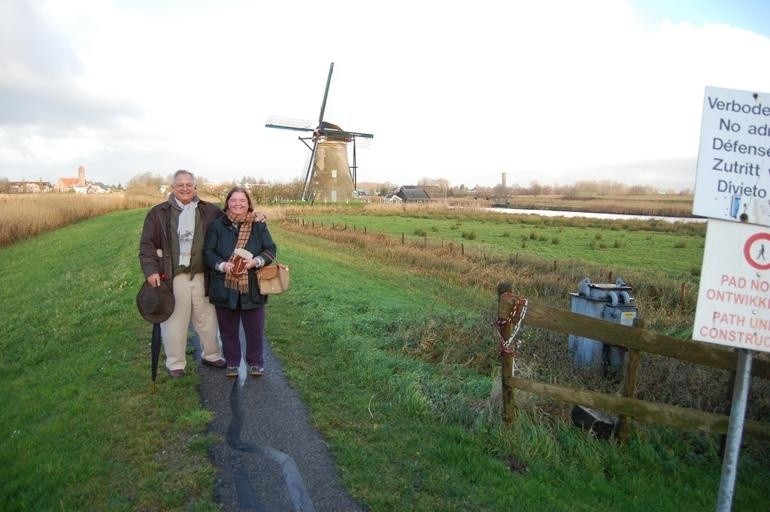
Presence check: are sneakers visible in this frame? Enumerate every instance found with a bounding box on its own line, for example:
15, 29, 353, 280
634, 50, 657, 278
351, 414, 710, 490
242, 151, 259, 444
170, 370, 188, 377
248, 364, 265, 376
198, 355, 225, 368
225, 367, 238, 378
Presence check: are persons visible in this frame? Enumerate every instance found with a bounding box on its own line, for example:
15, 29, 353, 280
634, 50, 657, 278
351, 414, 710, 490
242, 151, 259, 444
203, 185, 277, 379
137, 169, 267, 378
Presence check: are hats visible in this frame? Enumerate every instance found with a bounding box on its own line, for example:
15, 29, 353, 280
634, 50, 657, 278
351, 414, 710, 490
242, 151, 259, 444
136, 278, 176, 325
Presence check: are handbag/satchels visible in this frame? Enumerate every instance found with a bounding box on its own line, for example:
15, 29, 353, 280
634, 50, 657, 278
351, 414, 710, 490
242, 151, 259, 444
255, 264, 291, 295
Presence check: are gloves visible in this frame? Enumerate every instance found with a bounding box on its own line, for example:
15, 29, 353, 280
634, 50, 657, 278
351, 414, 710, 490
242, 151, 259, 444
228, 247, 254, 278
